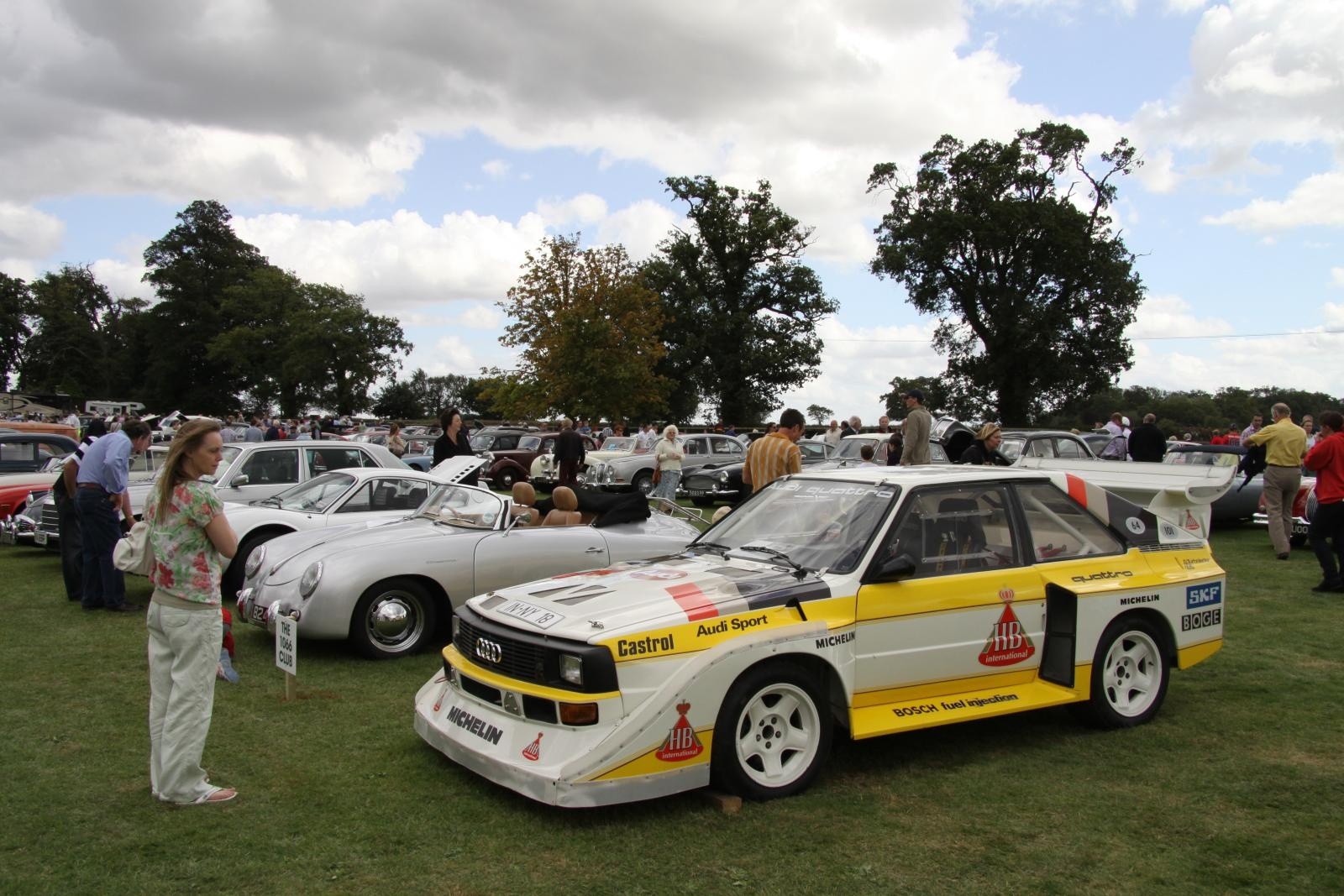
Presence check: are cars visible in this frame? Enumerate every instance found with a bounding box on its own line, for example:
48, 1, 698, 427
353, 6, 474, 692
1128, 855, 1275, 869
0, 415, 1318, 551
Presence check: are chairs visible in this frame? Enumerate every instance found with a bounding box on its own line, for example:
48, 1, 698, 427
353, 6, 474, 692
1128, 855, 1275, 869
509, 482, 581, 527
249, 453, 298, 481
372, 485, 428, 509
892, 498, 986, 573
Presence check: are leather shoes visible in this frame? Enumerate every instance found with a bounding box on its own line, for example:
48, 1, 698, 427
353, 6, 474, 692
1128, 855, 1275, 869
106, 601, 139, 610
82, 603, 104, 610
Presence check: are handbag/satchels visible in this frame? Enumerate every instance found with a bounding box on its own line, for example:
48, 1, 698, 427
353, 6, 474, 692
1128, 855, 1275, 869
113, 520, 155, 577
389, 436, 404, 456
652, 460, 661, 483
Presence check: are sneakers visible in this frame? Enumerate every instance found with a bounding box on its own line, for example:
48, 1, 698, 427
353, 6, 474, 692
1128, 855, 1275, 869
216, 649, 238, 684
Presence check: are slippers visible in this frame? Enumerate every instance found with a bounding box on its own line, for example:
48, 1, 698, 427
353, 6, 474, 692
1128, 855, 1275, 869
176, 785, 237, 805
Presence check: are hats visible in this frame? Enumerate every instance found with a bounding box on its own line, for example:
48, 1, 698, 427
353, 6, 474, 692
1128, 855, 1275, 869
1122, 416, 1130, 426
904, 390, 922, 400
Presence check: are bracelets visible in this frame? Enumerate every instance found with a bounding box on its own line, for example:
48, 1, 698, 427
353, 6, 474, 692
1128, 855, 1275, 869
127, 516, 133, 519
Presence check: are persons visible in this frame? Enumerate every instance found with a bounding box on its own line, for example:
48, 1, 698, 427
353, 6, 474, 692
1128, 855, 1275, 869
433, 408, 480, 487
859, 389, 932, 468
576, 420, 592, 434
1209, 403, 1344, 593
712, 423, 736, 444
220, 412, 365, 463
825, 415, 862, 447
956, 424, 1002, 568
596, 424, 624, 448
765, 422, 777, 434
1053, 411, 1193, 462
387, 423, 405, 458
55, 419, 150, 615
23, 411, 191, 443
552, 417, 585, 486
742, 408, 806, 493
142, 419, 239, 803
656, 425, 685, 513
638, 422, 658, 448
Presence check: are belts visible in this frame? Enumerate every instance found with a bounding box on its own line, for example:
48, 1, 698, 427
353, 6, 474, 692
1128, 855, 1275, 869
78, 483, 100, 488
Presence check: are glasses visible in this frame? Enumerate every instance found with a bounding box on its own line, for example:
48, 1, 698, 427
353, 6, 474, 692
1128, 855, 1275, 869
906, 396, 913, 400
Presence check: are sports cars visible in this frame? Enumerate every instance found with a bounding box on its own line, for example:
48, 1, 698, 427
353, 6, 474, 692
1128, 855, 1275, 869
236, 479, 705, 659
213, 453, 528, 596
411, 455, 1240, 812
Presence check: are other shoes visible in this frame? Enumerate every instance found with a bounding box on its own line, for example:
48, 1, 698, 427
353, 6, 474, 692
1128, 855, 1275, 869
1313, 576, 1343, 591
1278, 552, 1288, 560
665, 509, 677, 514
